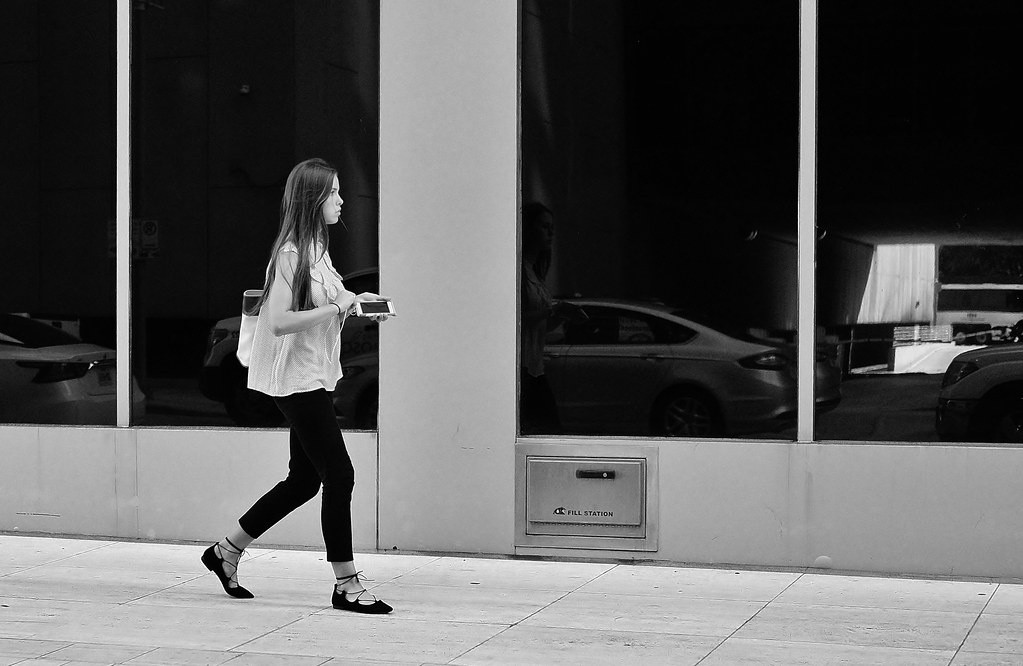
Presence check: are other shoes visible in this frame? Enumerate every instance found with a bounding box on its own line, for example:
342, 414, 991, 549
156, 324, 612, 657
331, 583, 393, 614
201, 542, 254, 599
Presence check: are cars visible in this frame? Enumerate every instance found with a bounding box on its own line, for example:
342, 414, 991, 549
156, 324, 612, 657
206, 261, 390, 413
772, 216, 1022, 445
1, 309, 146, 425
322, 295, 840, 442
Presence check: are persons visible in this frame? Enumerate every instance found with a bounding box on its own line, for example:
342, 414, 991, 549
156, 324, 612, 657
522, 202, 575, 435
202, 159, 394, 617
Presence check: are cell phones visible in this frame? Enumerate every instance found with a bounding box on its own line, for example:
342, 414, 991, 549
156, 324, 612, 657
356, 300, 397, 318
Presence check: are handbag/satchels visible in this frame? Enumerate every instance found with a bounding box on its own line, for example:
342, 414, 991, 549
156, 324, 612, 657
236, 289, 264, 368
